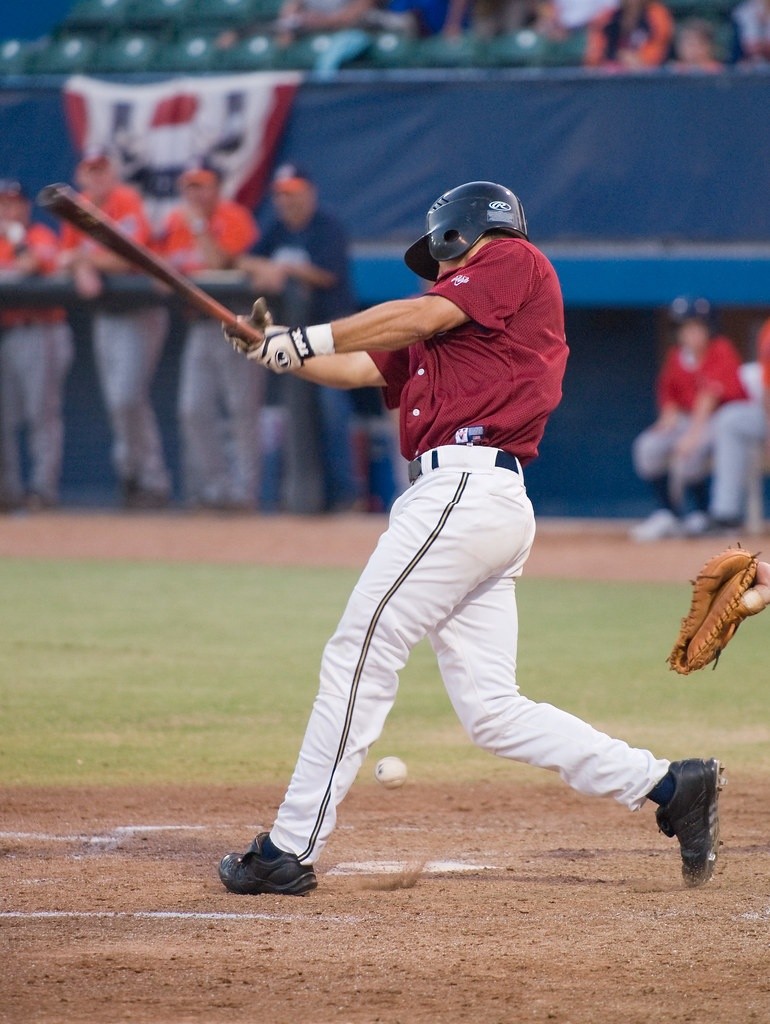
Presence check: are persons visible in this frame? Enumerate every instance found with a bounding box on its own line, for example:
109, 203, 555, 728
626, 292, 770, 544
212, 0, 770, 73
665, 541, 770, 676
221, 180, 727, 895
1, 145, 399, 516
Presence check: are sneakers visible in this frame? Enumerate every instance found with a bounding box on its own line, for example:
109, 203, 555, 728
219, 832, 318, 895
655, 757, 727, 888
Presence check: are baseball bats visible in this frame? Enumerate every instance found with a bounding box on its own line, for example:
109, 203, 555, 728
36, 182, 263, 345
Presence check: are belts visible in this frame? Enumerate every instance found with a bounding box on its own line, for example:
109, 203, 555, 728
415, 450, 518, 474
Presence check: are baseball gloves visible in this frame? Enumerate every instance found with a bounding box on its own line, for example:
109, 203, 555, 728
669, 548, 759, 676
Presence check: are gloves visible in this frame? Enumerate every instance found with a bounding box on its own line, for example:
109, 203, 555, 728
220, 312, 315, 375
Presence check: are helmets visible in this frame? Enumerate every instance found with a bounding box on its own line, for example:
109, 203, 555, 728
405, 181, 530, 282
666, 292, 721, 330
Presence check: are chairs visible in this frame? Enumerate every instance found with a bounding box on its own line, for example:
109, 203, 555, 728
0, 0, 744, 80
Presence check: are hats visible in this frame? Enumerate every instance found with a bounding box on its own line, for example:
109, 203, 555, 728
75, 137, 127, 173
0, 177, 27, 202
273, 164, 314, 195
178, 167, 220, 189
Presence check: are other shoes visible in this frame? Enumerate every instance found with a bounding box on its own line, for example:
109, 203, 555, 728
627, 506, 677, 542
705, 515, 741, 534
672, 510, 711, 534
